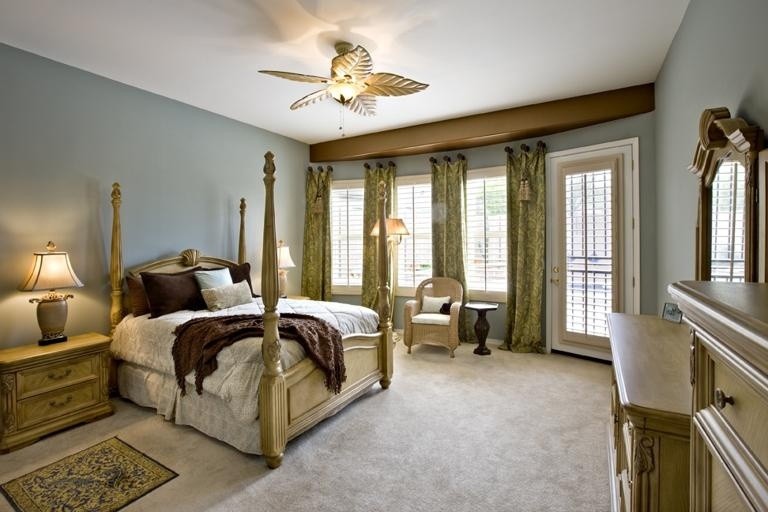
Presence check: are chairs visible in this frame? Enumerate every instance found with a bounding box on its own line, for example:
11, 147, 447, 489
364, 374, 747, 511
404, 277, 464, 358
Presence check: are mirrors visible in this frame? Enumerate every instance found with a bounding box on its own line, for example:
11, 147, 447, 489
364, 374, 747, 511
686, 107, 765, 282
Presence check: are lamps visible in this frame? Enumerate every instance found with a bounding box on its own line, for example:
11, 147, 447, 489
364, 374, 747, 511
277, 240, 296, 299
18, 241, 85, 345
370, 219, 410, 343
258, 42, 429, 118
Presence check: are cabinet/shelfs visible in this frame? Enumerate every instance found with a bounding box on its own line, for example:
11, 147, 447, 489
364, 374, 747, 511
667, 280, 768, 512
606, 312, 691, 511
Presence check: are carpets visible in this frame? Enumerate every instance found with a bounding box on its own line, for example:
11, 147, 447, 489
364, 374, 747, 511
0, 436, 179, 512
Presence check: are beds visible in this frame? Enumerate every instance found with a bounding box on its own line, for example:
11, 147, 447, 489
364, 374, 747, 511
110, 151, 393, 469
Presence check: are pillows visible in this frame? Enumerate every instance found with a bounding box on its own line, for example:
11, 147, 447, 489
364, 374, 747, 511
201, 279, 253, 312
193, 268, 233, 289
201, 263, 261, 298
125, 276, 150, 317
420, 295, 451, 313
139, 266, 201, 319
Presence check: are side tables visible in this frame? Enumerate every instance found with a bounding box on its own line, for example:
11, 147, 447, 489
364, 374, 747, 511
464, 302, 499, 355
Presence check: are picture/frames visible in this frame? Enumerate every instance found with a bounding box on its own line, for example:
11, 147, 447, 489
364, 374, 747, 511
661, 302, 682, 323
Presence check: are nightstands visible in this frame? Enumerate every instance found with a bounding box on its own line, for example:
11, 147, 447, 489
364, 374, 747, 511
0, 333, 115, 455
